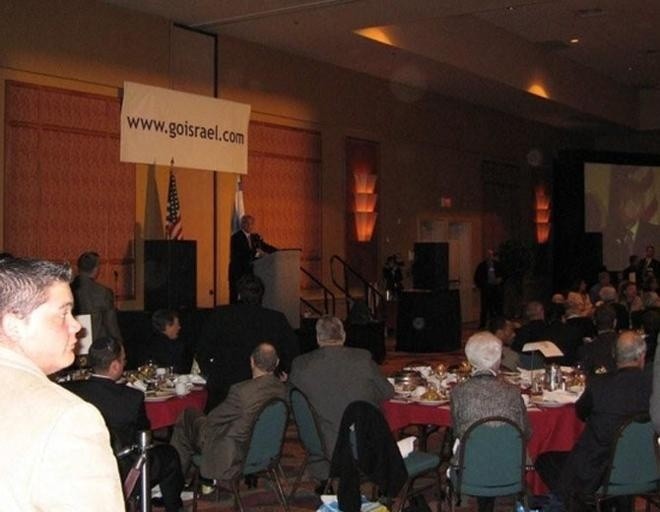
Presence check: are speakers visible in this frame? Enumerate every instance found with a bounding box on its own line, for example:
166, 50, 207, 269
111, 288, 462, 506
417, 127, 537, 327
413, 242, 448, 289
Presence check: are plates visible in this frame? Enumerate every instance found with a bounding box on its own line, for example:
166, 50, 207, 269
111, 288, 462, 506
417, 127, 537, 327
390, 364, 585, 408
124, 362, 203, 402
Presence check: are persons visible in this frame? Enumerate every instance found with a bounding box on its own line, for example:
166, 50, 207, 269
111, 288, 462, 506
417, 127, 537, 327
291, 246, 660, 510
228, 217, 278, 303
0, 251, 299, 510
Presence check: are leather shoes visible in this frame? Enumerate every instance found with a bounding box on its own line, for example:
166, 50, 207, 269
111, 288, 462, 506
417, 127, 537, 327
315, 484, 333, 494
245, 476, 257, 489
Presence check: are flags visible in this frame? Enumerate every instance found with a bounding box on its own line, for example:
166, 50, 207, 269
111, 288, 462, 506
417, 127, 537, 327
166, 169, 184, 243
231, 178, 245, 235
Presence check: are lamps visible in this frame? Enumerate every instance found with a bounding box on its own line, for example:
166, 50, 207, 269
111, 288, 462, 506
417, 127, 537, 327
352, 172, 380, 243
536, 194, 552, 243
